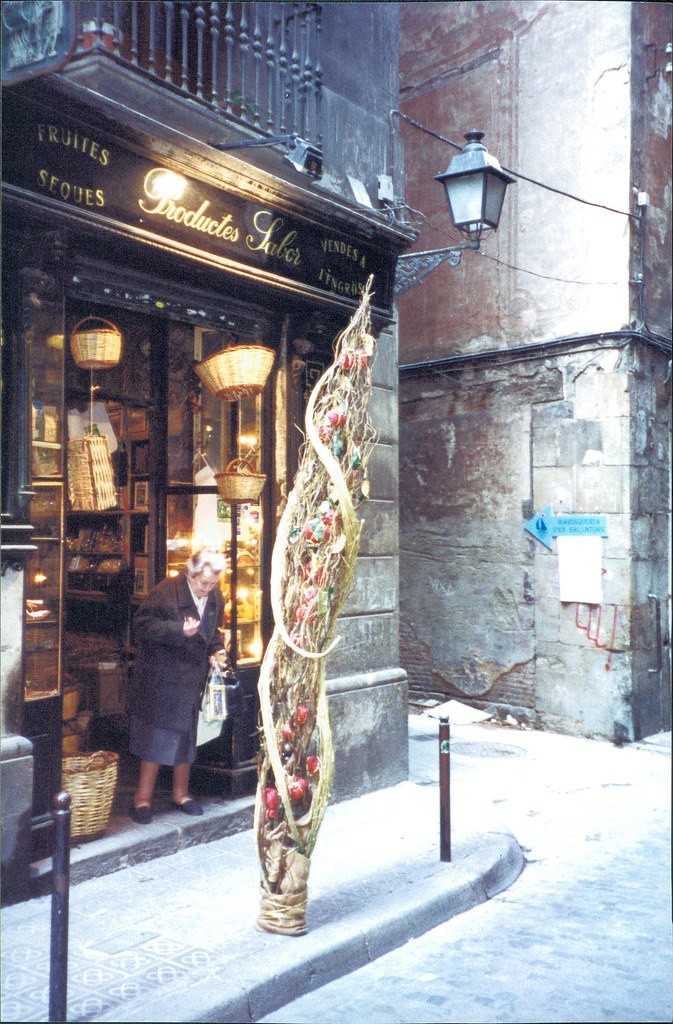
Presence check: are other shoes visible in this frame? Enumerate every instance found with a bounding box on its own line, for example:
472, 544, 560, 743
131, 801, 151, 824
171, 798, 204, 816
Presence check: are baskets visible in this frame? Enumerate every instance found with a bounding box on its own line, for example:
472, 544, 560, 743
193, 344, 276, 402
69, 434, 118, 512
70, 315, 122, 371
62, 751, 120, 837
213, 459, 267, 504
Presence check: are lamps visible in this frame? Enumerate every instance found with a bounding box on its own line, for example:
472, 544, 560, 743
393, 129, 519, 297
207, 132, 324, 180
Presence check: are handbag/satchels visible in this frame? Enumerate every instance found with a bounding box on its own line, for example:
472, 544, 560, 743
196, 661, 229, 747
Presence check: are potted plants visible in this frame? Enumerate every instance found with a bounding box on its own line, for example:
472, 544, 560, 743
217, 90, 257, 119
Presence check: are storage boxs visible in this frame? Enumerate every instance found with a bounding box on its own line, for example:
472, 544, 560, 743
69, 663, 130, 718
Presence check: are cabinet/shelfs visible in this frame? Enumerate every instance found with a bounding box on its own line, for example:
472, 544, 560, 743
64, 359, 193, 660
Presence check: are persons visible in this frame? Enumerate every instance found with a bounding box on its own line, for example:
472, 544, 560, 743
127, 546, 226, 824
